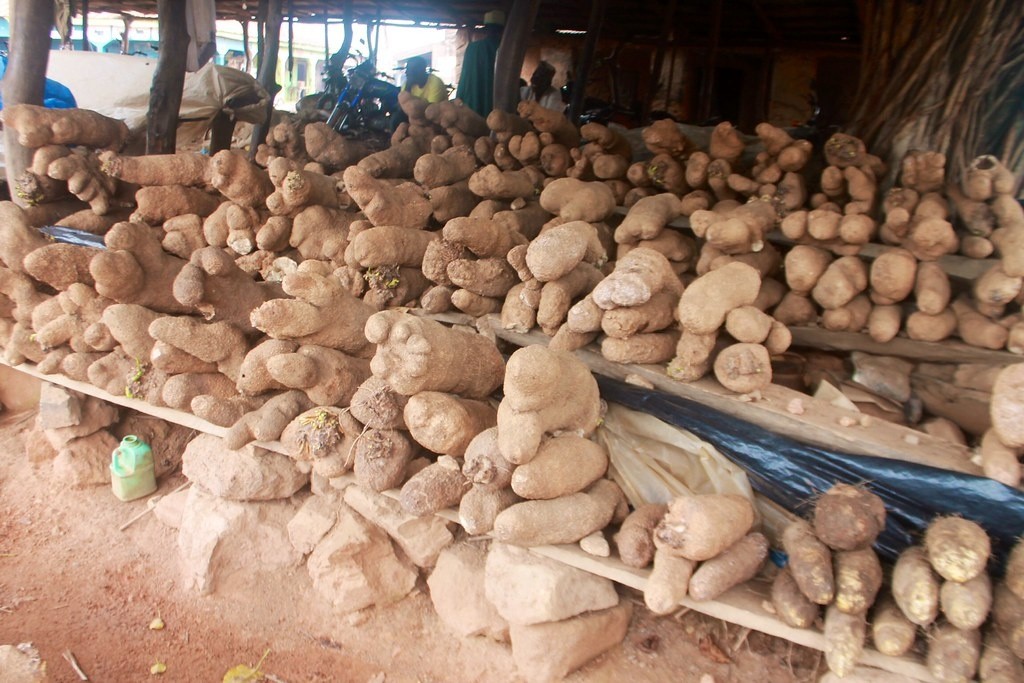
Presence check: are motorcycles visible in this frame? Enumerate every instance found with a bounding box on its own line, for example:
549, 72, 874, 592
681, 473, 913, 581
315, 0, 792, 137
319, 39, 455, 136
560, 70, 646, 126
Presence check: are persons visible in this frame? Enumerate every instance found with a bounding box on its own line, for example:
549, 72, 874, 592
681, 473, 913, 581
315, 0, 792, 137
519, 62, 565, 116
388, 55, 448, 132
456, 10, 507, 119
